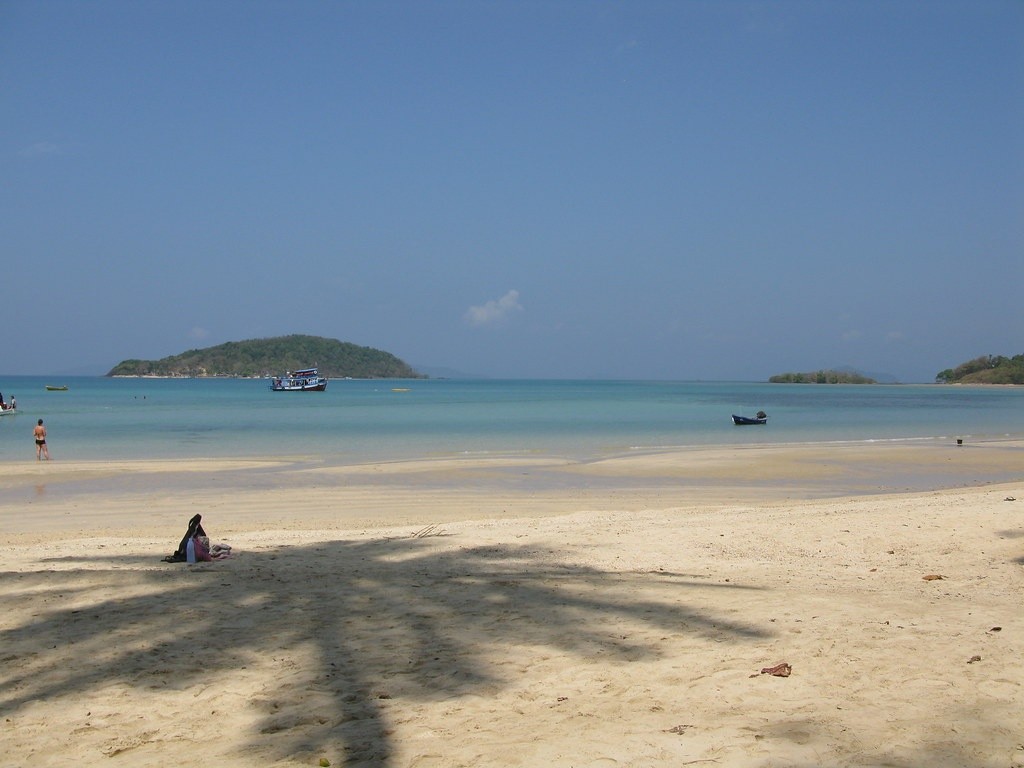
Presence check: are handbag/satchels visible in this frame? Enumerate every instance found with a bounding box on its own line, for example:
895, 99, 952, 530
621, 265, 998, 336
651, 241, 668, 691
161, 514, 206, 563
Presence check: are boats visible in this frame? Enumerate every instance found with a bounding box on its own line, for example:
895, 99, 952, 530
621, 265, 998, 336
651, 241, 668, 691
267, 368, 328, 391
0, 409, 13, 415
46, 385, 69, 391
731, 414, 767, 424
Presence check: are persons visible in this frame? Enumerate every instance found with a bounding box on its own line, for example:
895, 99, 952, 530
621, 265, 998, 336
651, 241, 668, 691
7, 395, 16, 409
0, 392, 3, 405
33, 419, 49, 460
